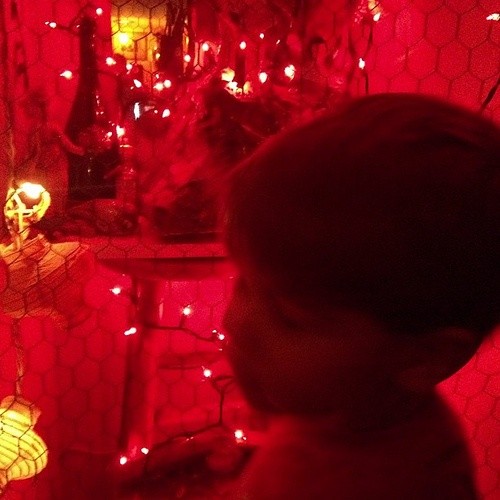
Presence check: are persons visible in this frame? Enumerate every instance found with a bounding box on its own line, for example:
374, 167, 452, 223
218, 88, 500, 500
